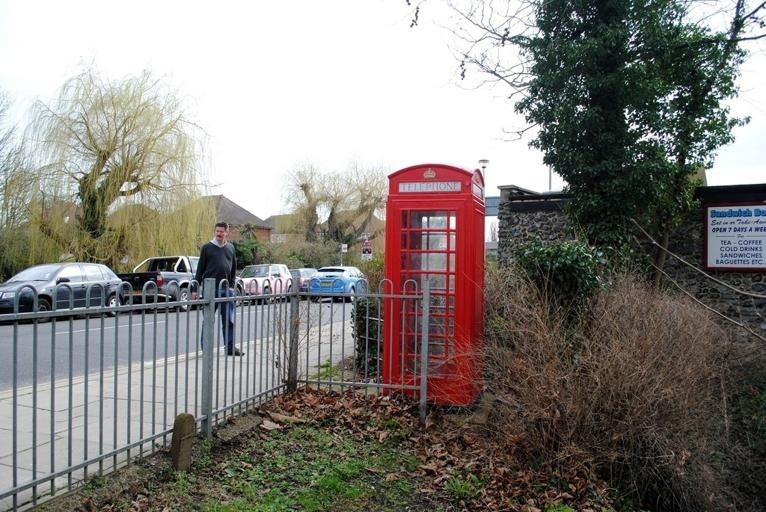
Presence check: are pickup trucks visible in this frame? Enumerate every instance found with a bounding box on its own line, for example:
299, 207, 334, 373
116, 255, 244, 313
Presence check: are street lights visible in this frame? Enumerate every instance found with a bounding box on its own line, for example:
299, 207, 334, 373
478, 159, 491, 179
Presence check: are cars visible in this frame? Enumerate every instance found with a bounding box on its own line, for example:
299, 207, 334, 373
0, 262, 125, 324
288, 268, 319, 296
307, 266, 369, 303
235, 263, 293, 305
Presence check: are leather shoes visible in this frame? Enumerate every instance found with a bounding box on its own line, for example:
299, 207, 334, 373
225, 348, 243, 356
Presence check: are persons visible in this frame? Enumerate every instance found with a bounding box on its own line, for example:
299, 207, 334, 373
191, 222, 245, 356
